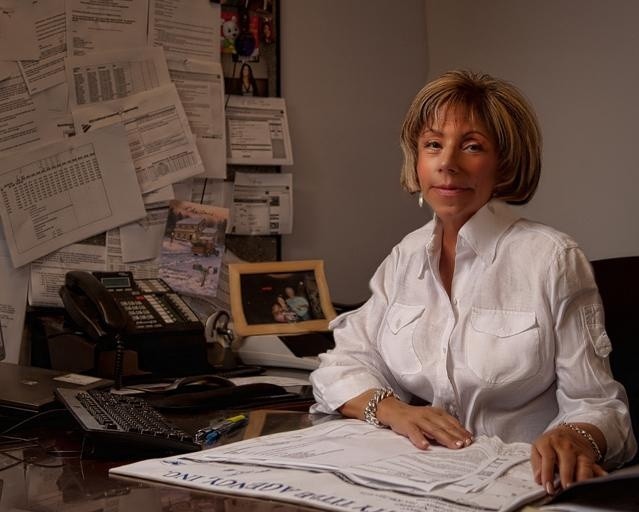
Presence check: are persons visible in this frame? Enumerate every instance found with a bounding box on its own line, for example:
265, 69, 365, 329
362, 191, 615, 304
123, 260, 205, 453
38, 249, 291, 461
273, 286, 311, 322
309, 68, 638, 495
235, 63, 259, 97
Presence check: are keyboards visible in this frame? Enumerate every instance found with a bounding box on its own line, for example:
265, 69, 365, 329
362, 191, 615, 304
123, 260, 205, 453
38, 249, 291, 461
55, 385, 201, 455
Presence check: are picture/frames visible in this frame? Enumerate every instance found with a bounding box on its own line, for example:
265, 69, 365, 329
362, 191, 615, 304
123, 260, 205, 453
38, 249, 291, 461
228, 259, 337, 337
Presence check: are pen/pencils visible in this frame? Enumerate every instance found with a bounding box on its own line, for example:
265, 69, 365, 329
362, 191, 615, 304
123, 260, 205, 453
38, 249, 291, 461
195, 415, 247, 445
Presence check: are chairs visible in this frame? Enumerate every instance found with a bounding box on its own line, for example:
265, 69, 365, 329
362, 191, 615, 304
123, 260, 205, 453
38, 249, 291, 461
591, 256, 637, 439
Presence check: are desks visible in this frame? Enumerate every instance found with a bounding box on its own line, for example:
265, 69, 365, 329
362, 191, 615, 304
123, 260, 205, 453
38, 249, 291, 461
0, 373, 638, 511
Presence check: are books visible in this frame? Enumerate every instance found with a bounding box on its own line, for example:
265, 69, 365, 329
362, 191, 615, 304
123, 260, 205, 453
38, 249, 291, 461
156, 200, 230, 299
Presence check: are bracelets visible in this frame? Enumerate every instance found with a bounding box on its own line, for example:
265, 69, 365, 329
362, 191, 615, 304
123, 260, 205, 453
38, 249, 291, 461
555, 421, 602, 463
364, 388, 400, 429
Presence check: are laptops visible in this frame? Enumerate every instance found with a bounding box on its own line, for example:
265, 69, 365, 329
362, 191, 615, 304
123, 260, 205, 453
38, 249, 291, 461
1, 323, 114, 414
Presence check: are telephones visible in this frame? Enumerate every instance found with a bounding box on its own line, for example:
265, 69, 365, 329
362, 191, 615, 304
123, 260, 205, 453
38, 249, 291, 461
58, 271, 205, 344
